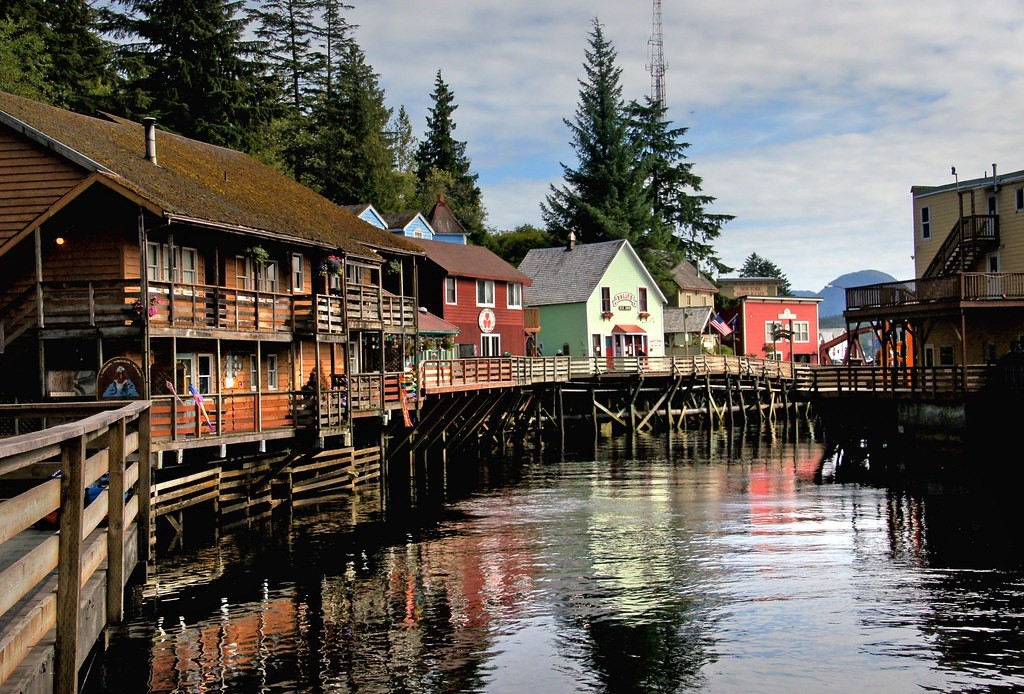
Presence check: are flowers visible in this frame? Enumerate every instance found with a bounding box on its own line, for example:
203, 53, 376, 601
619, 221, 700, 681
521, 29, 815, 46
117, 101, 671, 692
133, 294, 164, 318
318, 255, 345, 277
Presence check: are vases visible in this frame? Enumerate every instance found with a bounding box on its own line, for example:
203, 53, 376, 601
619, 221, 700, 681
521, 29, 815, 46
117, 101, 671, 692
603, 313, 613, 317
641, 313, 650, 317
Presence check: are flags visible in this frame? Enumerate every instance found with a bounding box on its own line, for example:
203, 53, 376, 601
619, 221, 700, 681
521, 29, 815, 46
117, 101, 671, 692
710, 315, 732, 336
733, 315, 739, 325
